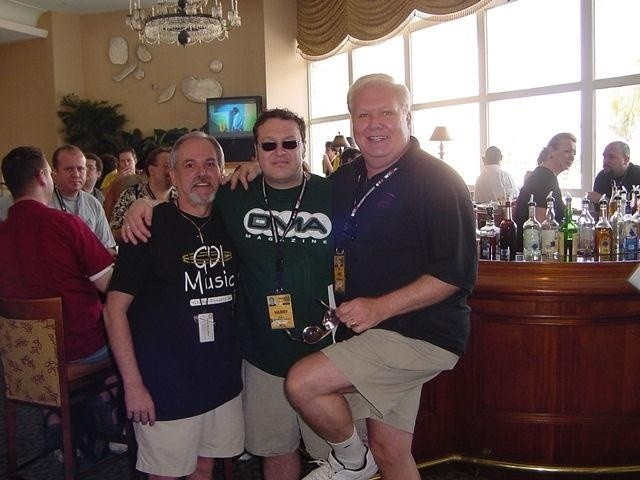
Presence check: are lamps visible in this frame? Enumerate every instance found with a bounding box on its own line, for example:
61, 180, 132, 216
330, 132, 349, 170
428, 127, 452, 162
123, 0, 240, 47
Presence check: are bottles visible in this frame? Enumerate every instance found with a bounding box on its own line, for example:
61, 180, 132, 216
471, 179, 640, 262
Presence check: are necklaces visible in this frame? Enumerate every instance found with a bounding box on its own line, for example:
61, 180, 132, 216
609, 173, 625, 189
176, 197, 213, 244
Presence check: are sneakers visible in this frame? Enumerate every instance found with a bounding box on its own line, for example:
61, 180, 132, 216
302, 444, 379, 480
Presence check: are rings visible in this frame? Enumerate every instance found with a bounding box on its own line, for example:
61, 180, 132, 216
351, 320, 358, 327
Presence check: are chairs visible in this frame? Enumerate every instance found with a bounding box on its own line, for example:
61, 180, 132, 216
1, 296, 137, 480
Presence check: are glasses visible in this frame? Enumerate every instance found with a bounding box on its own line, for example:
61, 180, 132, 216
260, 142, 298, 151
285, 300, 340, 344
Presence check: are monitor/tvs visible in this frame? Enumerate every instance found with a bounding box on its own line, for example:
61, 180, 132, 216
207, 96, 262, 141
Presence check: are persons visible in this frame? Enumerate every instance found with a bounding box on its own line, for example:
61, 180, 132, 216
0, 144, 178, 455
102, 132, 309, 480
341, 149, 361, 165
587, 142, 640, 212
475, 146, 520, 204
121, 109, 334, 480
220, 74, 478, 480
322, 142, 341, 178
515, 133, 576, 252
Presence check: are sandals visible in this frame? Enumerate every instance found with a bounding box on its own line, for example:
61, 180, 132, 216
47, 422, 84, 463
94, 390, 118, 428
